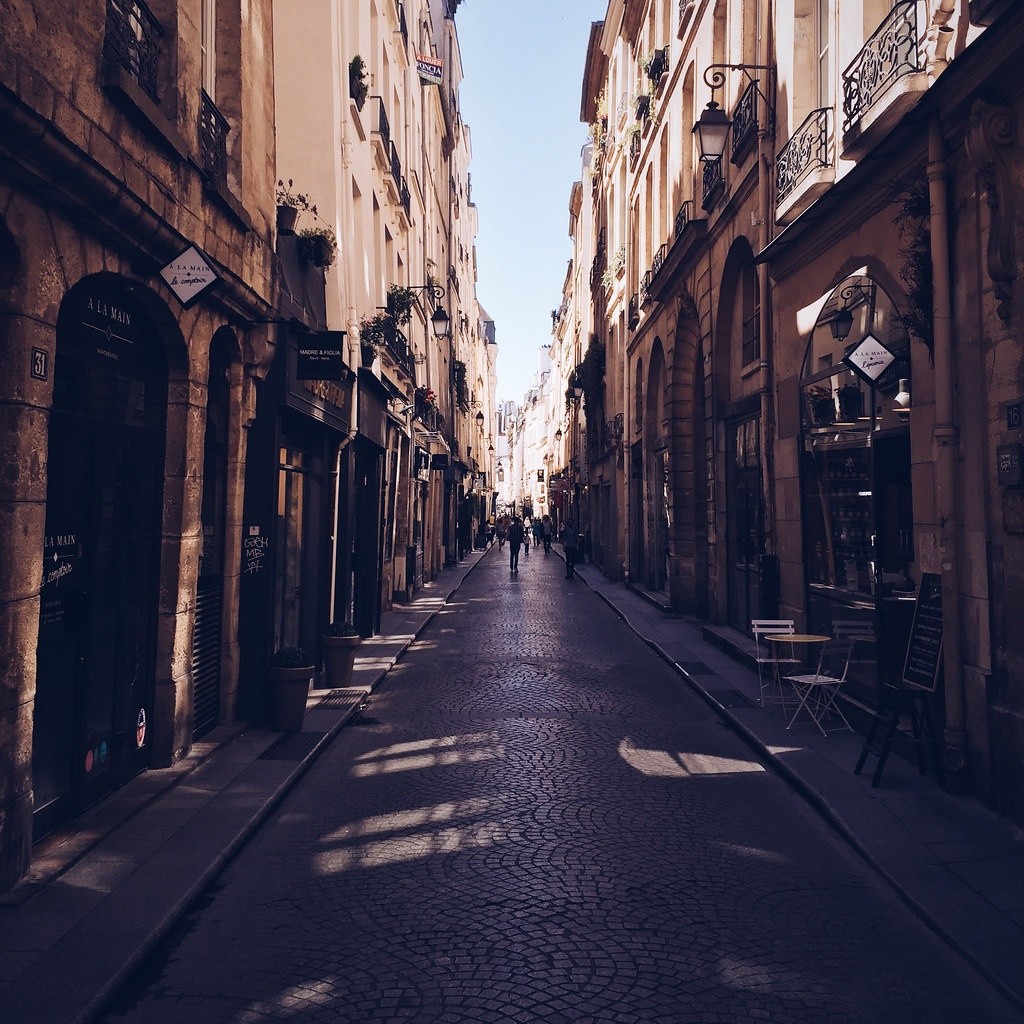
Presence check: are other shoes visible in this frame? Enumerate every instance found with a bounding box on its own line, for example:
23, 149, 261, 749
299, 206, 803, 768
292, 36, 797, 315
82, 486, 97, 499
565, 575, 573, 579
510, 570, 513, 573
514, 568, 519, 572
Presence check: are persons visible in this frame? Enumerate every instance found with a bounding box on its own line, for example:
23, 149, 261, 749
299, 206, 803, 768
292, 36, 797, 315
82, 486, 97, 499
485, 512, 577, 579
507, 518, 523, 573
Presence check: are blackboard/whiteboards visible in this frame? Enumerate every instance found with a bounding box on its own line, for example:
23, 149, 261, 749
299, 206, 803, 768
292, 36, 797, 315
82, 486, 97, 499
900, 571, 950, 694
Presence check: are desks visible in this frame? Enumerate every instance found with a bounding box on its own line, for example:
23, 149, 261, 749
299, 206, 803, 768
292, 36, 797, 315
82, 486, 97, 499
763, 634, 831, 643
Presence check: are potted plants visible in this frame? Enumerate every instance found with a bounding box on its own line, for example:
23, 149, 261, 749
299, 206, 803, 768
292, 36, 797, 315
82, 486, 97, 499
349, 55, 370, 112
299, 227, 340, 273
637, 48, 663, 79
632, 95, 649, 120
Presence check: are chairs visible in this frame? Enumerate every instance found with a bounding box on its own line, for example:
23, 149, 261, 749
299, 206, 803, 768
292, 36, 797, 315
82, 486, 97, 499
750, 619, 802, 709
782, 637, 856, 737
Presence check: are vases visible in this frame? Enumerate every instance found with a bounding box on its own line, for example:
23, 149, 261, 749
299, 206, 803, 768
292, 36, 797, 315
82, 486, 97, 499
277, 205, 298, 230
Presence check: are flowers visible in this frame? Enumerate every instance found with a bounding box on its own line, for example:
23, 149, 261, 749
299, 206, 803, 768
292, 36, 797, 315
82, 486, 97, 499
276, 179, 318, 221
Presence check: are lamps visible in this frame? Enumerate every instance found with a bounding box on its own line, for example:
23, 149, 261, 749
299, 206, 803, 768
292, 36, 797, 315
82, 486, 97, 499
574, 378, 585, 400
555, 429, 562, 441
405, 285, 451, 340
543, 454, 548, 464
498, 461, 503, 476
464, 400, 485, 426
480, 437, 494, 456
827, 285, 877, 342
